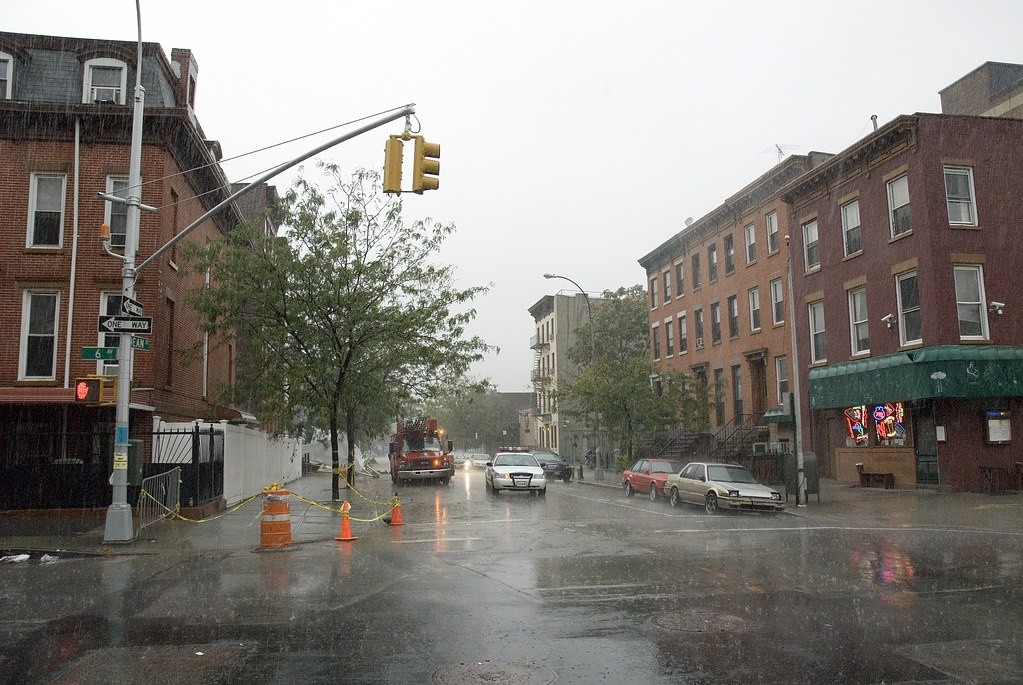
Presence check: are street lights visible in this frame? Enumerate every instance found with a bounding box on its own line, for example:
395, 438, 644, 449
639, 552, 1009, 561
544, 273, 600, 467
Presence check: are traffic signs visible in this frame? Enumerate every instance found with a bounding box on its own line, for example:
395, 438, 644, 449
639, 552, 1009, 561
79, 295, 153, 361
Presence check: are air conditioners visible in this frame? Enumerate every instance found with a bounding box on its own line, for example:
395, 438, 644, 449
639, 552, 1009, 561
697, 338, 703, 347
770, 442, 787, 453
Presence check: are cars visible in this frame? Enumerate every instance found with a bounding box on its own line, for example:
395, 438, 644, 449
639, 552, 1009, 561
664, 462, 786, 516
468, 453, 490, 471
363, 450, 377, 458
485, 452, 546, 495
451, 451, 466, 471
622, 458, 685, 502
531, 451, 572, 482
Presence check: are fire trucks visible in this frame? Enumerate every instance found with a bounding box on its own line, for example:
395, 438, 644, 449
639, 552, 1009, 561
388, 418, 453, 485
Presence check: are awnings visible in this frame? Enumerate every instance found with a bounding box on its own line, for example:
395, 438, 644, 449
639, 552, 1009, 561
809, 345, 1023, 410
763, 407, 793, 423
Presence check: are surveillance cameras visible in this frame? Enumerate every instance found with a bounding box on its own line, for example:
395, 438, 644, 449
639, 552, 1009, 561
990, 301, 1006, 309
881, 314, 893, 323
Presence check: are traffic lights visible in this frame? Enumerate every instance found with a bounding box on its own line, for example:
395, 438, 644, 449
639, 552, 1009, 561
413, 138, 441, 195
74, 378, 102, 402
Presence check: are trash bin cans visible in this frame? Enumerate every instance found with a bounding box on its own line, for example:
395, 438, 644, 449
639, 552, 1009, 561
785, 449, 821, 494
980, 466, 1005, 494
742, 453, 785, 485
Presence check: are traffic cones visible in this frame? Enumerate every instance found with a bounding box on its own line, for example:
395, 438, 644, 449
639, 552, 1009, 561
334, 501, 358, 540
389, 492, 405, 525
